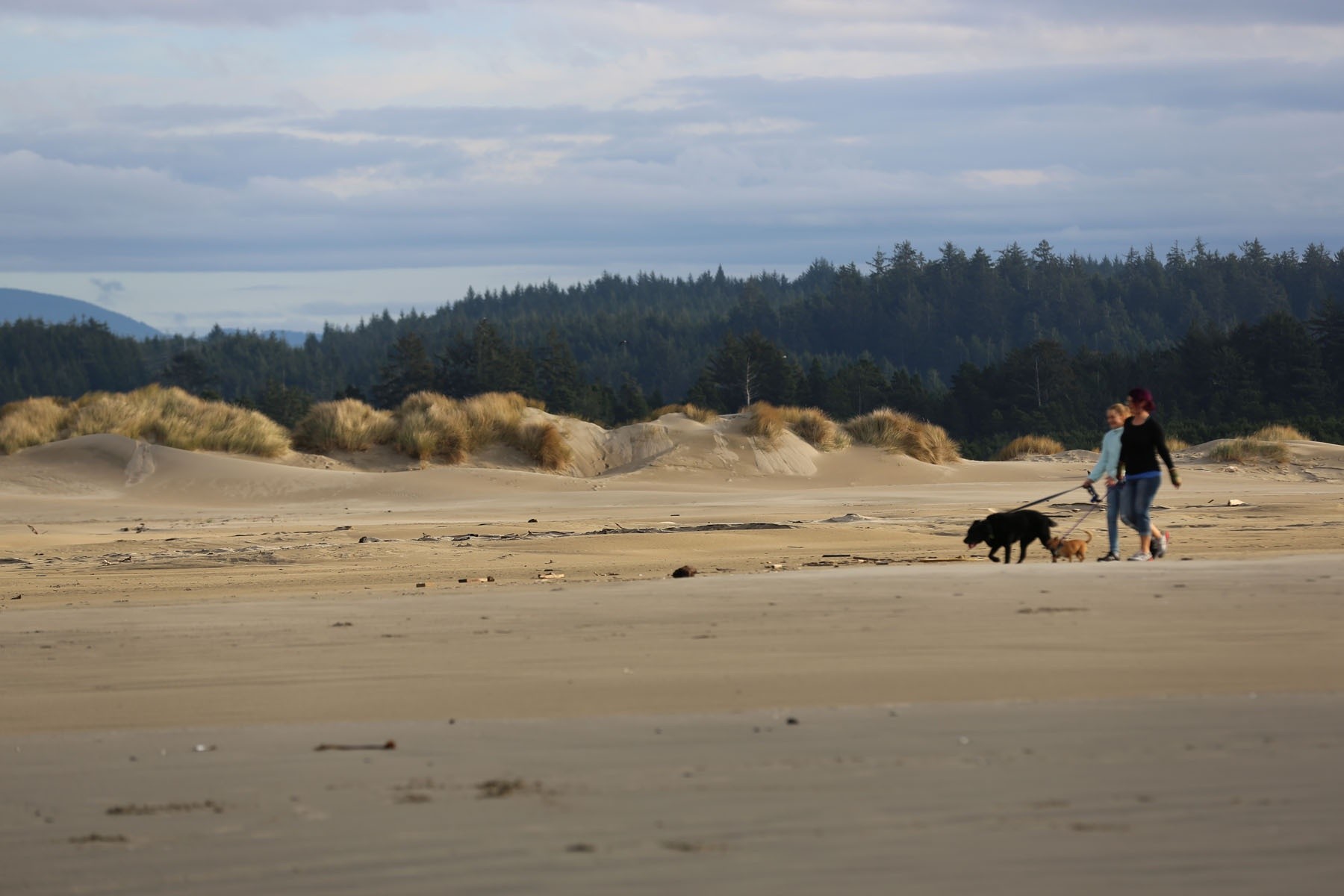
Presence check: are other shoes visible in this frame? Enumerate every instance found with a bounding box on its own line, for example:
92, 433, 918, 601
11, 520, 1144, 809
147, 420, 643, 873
1098, 551, 1120, 562
1149, 536, 1157, 559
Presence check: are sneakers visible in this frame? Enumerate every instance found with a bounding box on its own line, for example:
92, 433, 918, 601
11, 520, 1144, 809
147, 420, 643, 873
1127, 551, 1153, 562
1156, 531, 1169, 557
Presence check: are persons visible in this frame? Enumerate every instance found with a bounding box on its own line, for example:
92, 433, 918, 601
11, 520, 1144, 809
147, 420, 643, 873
1083, 403, 1161, 562
1116, 388, 1182, 561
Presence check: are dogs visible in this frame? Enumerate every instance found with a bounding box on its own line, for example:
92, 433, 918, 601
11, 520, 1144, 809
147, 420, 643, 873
962, 508, 1057, 564
1046, 528, 1094, 561
